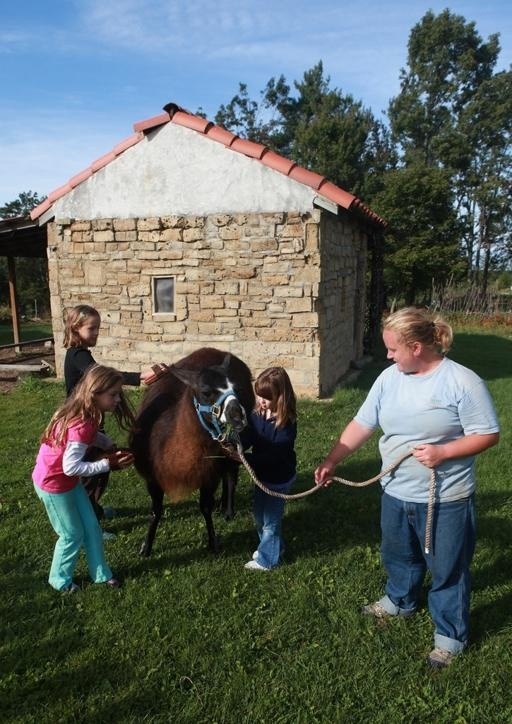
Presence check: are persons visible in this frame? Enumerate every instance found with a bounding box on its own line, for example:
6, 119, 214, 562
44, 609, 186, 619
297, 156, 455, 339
229, 366, 298, 572
313, 306, 501, 669
63, 303, 167, 541
31, 365, 135, 596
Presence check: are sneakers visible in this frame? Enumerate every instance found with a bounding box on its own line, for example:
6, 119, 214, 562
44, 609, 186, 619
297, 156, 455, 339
102, 530, 117, 543
242, 545, 286, 571
105, 578, 122, 588
427, 647, 459, 669
102, 507, 114, 516
363, 601, 392, 619
63, 583, 81, 594
